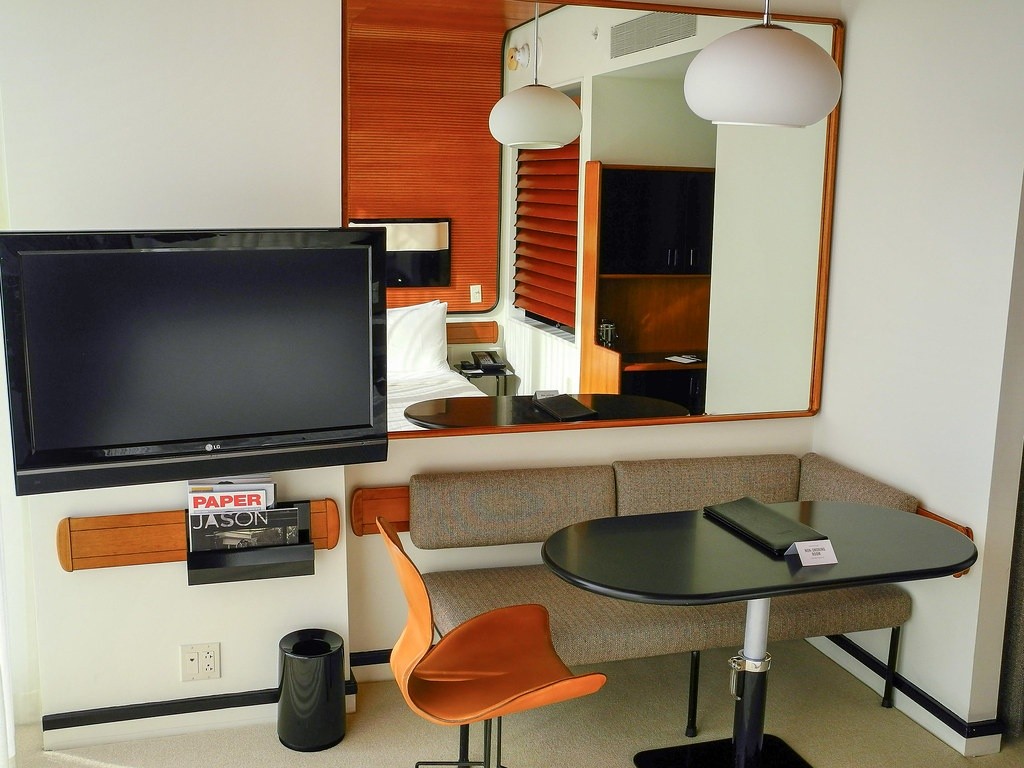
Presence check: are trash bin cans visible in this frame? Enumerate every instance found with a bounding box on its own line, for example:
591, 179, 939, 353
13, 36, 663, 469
277, 628, 347, 752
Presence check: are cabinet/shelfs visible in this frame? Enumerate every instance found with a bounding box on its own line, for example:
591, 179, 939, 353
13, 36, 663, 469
580, 161, 715, 417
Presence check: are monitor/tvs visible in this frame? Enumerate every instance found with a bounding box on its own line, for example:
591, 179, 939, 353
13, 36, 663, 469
0, 227, 388, 496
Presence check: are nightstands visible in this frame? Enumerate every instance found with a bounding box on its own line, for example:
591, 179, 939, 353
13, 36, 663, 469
454, 364, 514, 396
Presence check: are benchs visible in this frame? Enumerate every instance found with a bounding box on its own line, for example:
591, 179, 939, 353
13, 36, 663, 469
351, 453, 973, 738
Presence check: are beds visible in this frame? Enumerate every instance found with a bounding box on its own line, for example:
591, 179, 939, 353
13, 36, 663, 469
387, 300, 489, 432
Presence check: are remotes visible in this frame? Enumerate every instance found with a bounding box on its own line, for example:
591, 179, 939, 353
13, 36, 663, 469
461, 360, 476, 369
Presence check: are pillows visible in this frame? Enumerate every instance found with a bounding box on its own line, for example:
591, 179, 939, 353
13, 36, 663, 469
387, 302, 450, 381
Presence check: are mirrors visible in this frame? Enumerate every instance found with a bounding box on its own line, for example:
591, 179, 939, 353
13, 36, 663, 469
343, 0, 847, 439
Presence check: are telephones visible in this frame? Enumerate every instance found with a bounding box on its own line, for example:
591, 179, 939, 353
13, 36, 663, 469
472, 351, 506, 374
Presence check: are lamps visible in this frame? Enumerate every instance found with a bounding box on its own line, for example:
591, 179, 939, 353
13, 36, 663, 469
684, 0, 842, 128
489, 0, 583, 150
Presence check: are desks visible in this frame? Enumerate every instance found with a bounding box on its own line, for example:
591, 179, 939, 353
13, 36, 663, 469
404, 394, 691, 429
541, 501, 978, 768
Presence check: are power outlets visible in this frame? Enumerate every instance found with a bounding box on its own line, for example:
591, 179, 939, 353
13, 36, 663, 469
180, 643, 221, 681
470, 285, 482, 303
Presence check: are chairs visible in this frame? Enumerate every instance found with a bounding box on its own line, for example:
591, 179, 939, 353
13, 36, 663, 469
376, 517, 606, 768
